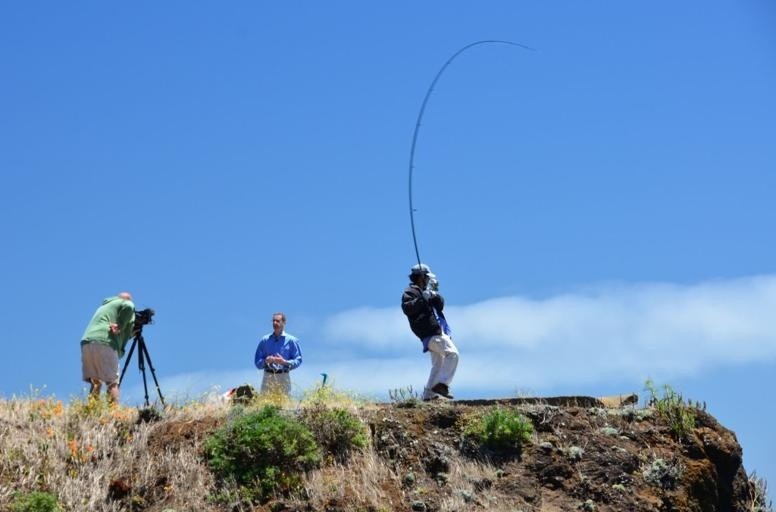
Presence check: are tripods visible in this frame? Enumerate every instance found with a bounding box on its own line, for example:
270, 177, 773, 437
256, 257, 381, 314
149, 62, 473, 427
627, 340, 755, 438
118, 331, 164, 407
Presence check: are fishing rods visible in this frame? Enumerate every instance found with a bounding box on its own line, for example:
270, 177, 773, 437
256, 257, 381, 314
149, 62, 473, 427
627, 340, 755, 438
408, 39, 538, 291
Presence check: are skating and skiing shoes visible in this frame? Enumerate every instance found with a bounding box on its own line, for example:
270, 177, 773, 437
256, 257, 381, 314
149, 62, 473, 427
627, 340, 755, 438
432, 382, 453, 399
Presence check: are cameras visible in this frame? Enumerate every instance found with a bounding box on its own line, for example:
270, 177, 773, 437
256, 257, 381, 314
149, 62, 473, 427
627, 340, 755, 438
135, 308, 152, 324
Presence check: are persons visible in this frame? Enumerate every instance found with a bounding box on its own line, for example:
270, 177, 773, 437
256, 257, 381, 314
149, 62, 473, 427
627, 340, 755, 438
253, 312, 304, 397
400, 263, 460, 401
80, 292, 138, 407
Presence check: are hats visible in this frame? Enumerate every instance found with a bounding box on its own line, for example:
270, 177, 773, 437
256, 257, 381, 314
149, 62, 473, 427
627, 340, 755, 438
410, 263, 435, 279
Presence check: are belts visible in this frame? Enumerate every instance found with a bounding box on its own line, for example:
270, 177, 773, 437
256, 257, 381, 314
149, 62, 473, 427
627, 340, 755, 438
264, 368, 289, 375
